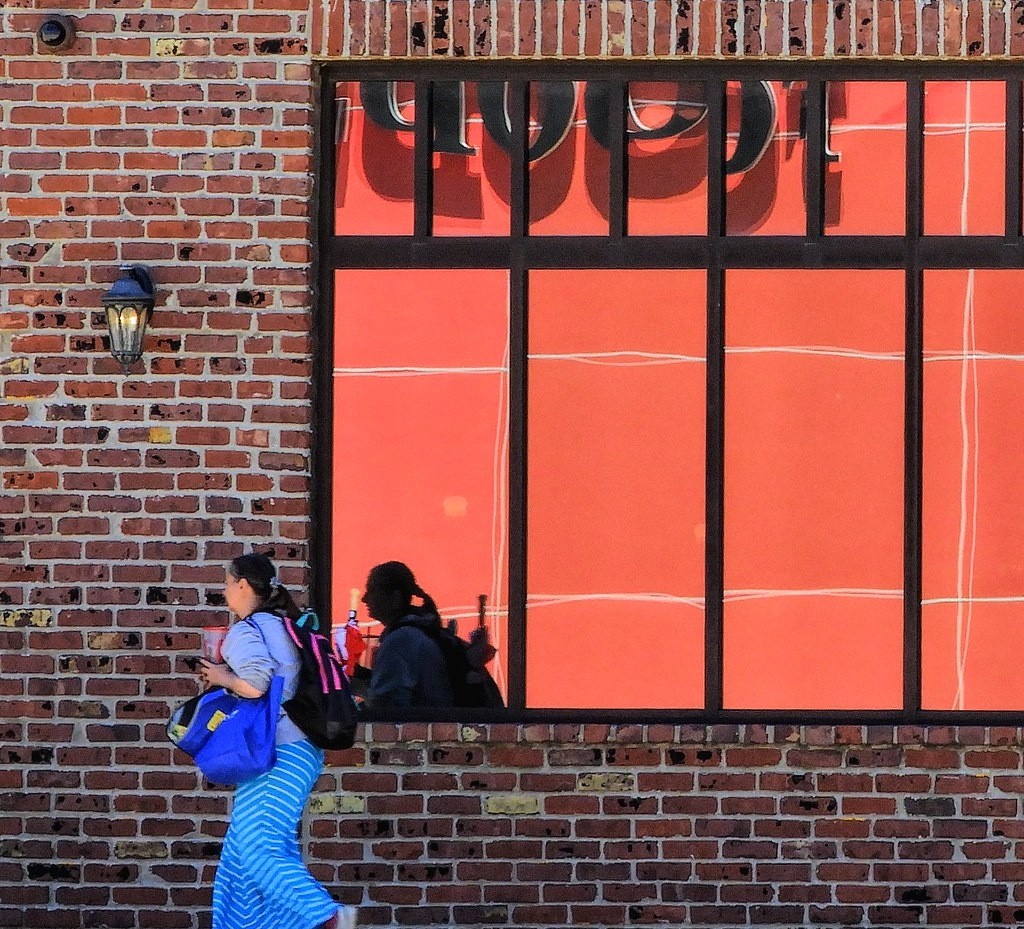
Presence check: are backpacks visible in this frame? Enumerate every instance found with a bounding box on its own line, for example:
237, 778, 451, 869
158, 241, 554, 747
375, 620, 510, 717
249, 608, 355, 750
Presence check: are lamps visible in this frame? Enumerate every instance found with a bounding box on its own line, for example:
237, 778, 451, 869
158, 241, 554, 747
101, 262, 156, 365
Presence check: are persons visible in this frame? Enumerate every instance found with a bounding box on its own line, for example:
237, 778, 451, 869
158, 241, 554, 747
350, 560, 459, 712
199, 553, 358, 929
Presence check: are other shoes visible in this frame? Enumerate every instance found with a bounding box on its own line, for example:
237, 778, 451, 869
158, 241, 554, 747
335, 907, 358, 929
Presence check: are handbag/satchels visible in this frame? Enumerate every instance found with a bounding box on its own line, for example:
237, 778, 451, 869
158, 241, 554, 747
166, 620, 283, 786
